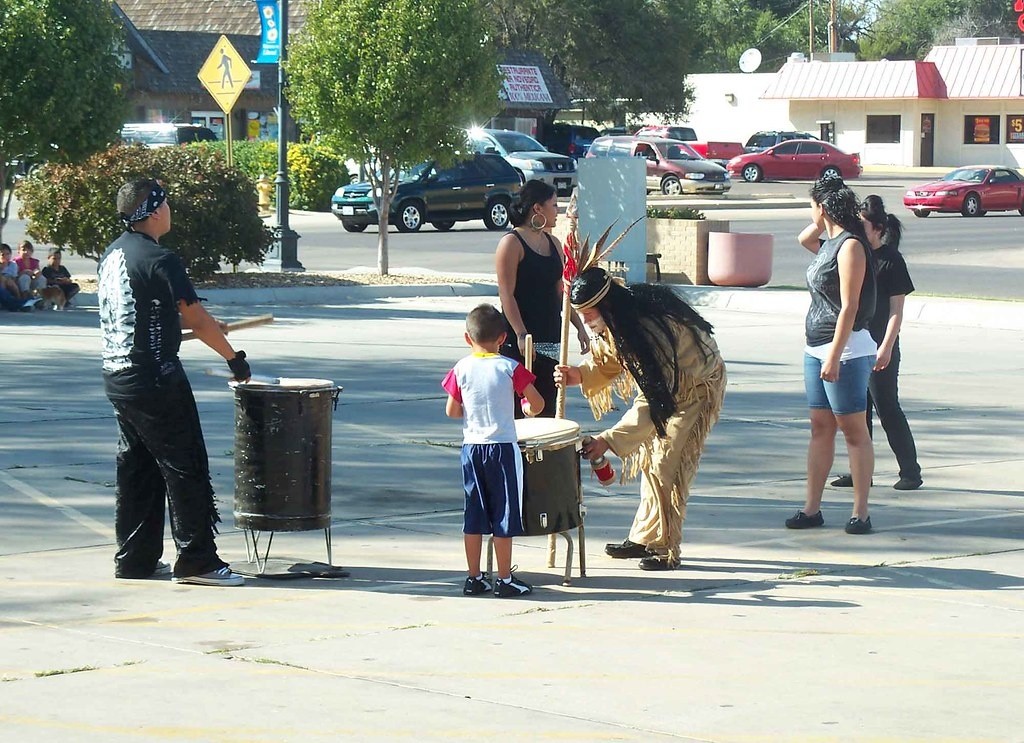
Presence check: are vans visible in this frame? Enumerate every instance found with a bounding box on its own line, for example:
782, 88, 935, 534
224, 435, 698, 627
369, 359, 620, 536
584, 136, 732, 196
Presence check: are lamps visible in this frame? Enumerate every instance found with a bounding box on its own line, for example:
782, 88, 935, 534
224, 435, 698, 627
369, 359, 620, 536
725, 93, 733, 102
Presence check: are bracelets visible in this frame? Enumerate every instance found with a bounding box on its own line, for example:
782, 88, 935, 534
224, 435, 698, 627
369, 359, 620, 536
516, 332, 530, 339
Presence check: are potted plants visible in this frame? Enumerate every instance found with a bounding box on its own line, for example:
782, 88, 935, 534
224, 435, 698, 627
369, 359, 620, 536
646, 205, 731, 286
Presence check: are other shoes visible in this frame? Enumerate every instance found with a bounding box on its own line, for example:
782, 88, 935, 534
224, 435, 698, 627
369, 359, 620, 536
830, 474, 873, 487
893, 478, 924, 490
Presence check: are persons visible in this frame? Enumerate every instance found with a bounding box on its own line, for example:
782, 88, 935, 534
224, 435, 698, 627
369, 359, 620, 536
552, 267, 728, 572
786, 175, 875, 533
0, 239, 43, 311
441, 304, 545, 597
495, 179, 590, 420
798, 197, 923, 490
97, 179, 251, 587
41, 247, 80, 306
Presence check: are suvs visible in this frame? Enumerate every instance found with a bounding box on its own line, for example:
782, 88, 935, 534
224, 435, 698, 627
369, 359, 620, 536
744, 130, 825, 154
553, 124, 602, 159
331, 154, 522, 233
120, 123, 217, 148
468, 129, 579, 196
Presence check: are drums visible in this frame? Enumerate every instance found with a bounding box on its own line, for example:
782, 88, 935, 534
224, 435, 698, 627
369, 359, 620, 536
230, 378, 335, 531
488, 419, 584, 537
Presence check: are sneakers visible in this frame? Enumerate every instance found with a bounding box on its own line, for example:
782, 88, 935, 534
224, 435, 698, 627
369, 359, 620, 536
638, 555, 682, 571
785, 510, 825, 528
604, 538, 659, 558
495, 565, 533, 597
172, 568, 245, 586
152, 560, 171, 575
845, 515, 872, 534
463, 572, 493, 595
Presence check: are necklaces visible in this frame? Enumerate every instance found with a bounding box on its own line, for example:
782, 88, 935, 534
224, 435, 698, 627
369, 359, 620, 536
531, 239, 542, 255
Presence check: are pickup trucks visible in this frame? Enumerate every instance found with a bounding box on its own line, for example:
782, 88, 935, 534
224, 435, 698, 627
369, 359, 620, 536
635, 125, 744, 169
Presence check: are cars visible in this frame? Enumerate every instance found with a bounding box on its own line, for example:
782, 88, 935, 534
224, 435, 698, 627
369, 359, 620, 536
599, 126, 630, 137
726, 139, 863, 182
903, 165, 1024, 218
